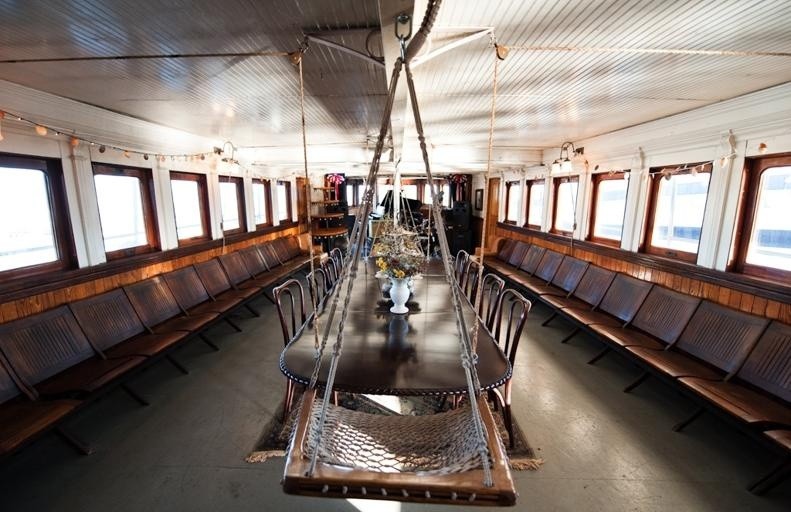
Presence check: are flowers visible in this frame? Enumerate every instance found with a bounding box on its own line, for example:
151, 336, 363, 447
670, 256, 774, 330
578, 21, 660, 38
374, 238, 423, 276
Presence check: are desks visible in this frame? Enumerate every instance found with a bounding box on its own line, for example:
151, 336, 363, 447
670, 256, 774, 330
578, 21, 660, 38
278, 253, 512, 415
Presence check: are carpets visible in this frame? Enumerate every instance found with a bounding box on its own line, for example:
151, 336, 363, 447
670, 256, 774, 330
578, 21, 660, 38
244, 383, 543, 471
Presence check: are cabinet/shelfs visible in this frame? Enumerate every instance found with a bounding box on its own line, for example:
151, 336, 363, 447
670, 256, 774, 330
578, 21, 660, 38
310, 187, 350, 238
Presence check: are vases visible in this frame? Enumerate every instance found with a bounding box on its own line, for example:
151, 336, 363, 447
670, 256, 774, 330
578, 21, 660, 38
381, 280, 414, 313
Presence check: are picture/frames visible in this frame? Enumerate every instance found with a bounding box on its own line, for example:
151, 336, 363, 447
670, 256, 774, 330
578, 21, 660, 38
475, 189, 483, 211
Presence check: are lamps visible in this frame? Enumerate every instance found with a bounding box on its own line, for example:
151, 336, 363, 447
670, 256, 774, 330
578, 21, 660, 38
551, 141, 584, 174
213, 140, 239, 165
